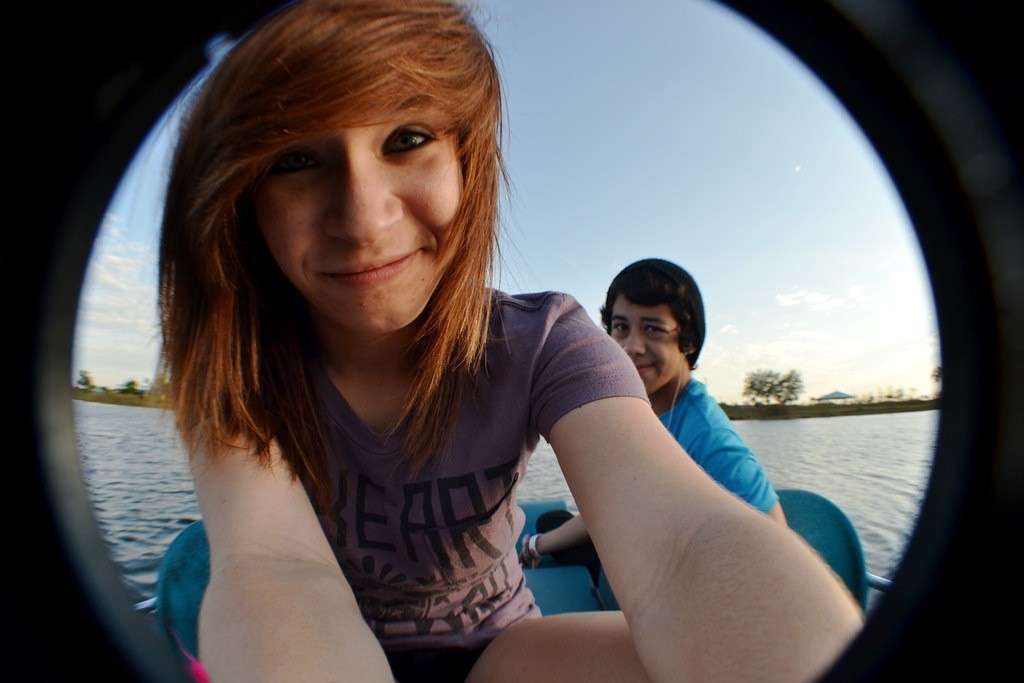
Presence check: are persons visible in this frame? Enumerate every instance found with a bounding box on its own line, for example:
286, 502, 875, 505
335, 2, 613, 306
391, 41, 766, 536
157, 0, 865, 683
518, 258, 790, 591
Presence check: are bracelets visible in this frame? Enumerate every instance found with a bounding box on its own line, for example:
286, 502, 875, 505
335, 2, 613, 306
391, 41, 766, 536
522, 532, 543, 567
